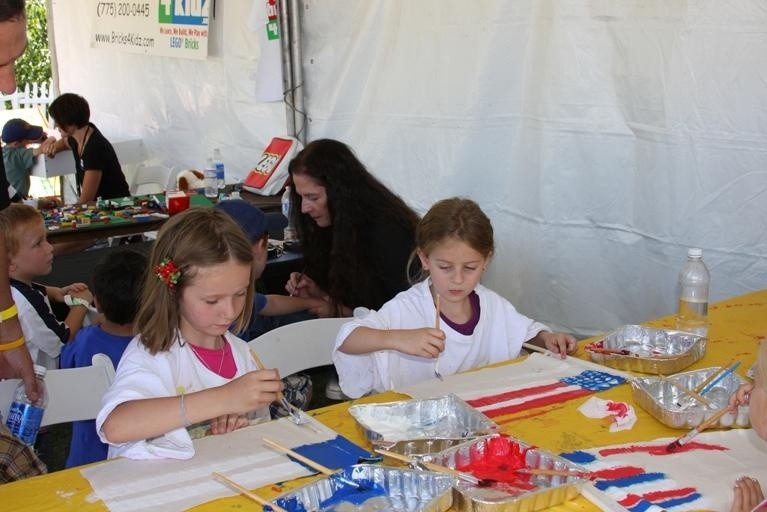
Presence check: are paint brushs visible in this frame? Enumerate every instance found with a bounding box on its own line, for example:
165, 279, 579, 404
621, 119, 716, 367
289, 263, 308, 300
591, 349, 633, 355
377, 450, 496, 488
676, 358, 733, 406
645, 354, 691, 359
435, 294, 446, 382
657, 371, 719, 411
212, 471, 288, 512
698, 358, 743, 404
249, 349, 304, 423
668, 390, 750, 450
264, 437, 370, 493
516, 467, 592, 483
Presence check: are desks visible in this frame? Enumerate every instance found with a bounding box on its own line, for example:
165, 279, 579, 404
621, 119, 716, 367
27, 135, 145, 206
0, 287, 766, 512
43, 182, 283, 245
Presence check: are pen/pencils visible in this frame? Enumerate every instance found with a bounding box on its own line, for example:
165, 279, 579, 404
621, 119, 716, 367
152, 195, 167, 212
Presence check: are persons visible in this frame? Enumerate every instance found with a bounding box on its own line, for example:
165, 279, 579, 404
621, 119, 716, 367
215, 198, 327, 342
0, 118, 55, 203
285, 139, 428, 318
46, 93, 132, 203
728, 334, 767, 511
332, 196, 579, 399
58, 246, 148, 468
96, 209, 286, 459
0, 1, 48, 483
3, 203, 93, 373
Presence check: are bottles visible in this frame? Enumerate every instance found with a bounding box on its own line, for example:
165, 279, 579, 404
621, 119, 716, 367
214, 146, 225, 189
204, 157, 218, 196
281, 185, 292, 217
6, 363, 48, 454
676, 247, 710, 340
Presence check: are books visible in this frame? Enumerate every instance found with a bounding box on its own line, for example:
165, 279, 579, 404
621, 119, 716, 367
241, 133, 303, 196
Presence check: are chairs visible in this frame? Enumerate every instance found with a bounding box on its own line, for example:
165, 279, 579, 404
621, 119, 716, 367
1, 352, 117, 431
245, 305, 370, 381
107, 162, 179, 247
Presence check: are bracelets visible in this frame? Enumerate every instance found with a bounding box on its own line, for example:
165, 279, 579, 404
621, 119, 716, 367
0, 303, 18, 322
178, 394, 192, 427
0, 335, 26, 352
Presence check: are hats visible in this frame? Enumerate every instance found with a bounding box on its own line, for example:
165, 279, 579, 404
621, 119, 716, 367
215, 198, 289, 243
2, 118, 44, 142
